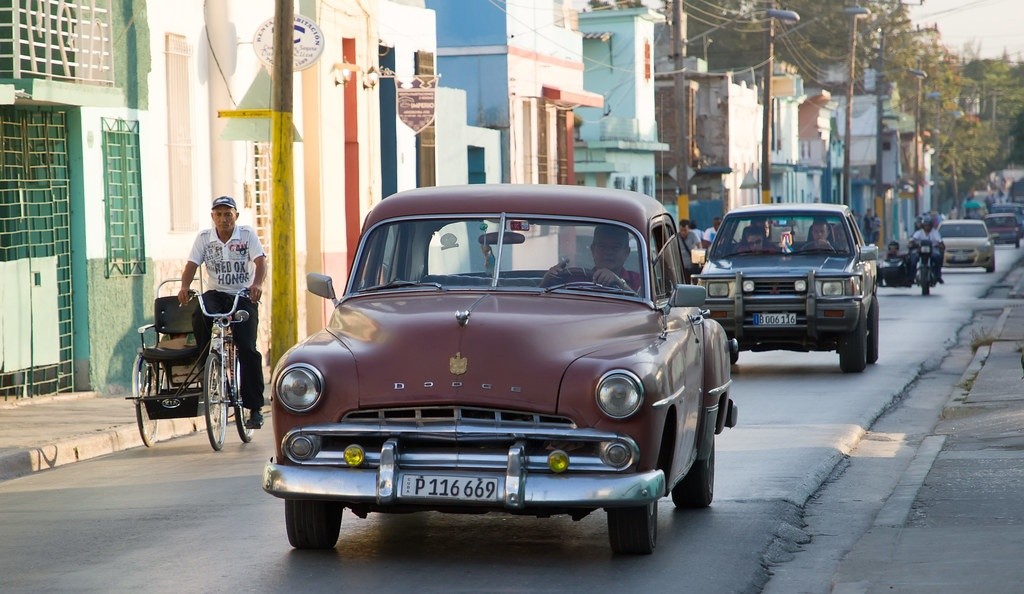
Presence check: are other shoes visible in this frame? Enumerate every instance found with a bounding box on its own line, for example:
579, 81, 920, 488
938, 277, 943, 284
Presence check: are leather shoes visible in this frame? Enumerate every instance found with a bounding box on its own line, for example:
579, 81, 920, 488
248, 414, 264, 428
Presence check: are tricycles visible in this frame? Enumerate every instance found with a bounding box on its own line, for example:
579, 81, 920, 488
124, 275, 263, 451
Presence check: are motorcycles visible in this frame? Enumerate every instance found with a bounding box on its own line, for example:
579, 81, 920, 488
873, 234, 944, 296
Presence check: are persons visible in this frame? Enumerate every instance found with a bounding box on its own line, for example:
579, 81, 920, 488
885, 240, 905, 259
735, 224, 780, 256
851, 208, 881, 246
677, 217, 722, 285
538, 225, 642, 298
938, 206, 983, 220
179, 196, 268, 427
798, 217, 849, 254
1006, 218, 1013, 224
956, 227, 970, 236
908, 210, 944, 284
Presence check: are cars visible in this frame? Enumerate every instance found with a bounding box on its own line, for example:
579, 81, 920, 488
260, 183, 738, 558
936, 219, 1001, 273
990, 203, 1024, 239
690, 202, 881, 374
980, 213, 1023, 248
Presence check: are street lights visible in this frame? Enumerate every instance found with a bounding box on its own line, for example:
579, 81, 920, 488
842, 67, 928, 205
875, 91, 943, 245
671, 8, 799, 197
913, 110, 965, 216
761, 6, 871, 203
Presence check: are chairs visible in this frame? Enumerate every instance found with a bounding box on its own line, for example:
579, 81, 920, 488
417, 271, 542, 285
138, 277, 209, 363
741, 226, 771, 247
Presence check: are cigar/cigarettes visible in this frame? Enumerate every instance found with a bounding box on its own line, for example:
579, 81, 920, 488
562, 257, 568, 266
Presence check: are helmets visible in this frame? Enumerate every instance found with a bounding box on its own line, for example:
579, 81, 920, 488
914, 216, 922, 224
922, 215, 932, 224
888, 241, 899, 250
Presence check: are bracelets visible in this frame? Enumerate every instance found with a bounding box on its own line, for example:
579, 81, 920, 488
619, 278, 627, 290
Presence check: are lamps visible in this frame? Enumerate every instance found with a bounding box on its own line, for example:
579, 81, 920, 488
363, 61, 380, 90
334, 57, 351, 86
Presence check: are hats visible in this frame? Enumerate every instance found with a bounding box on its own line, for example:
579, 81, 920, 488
212, 196, 236, 210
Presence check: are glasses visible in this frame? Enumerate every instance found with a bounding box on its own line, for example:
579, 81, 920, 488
748, 239, 762, 246
